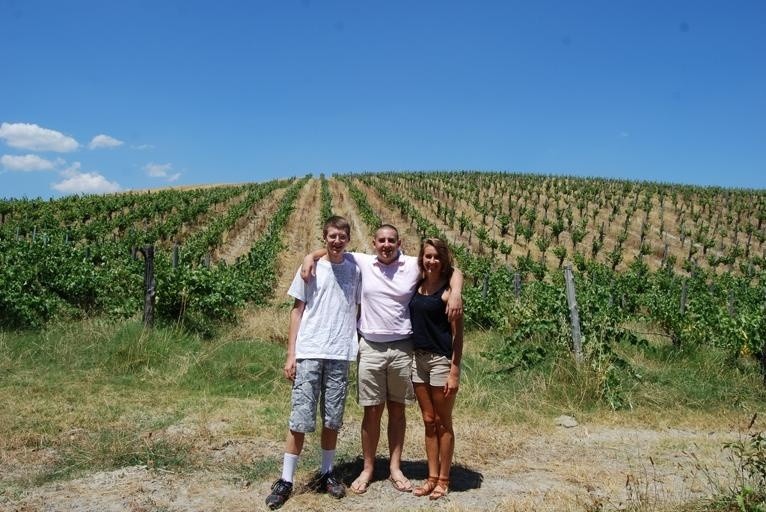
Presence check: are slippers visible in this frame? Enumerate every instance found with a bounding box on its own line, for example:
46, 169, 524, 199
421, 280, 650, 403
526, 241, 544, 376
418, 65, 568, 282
350, 478, 373, 494
390, 471, 413, 492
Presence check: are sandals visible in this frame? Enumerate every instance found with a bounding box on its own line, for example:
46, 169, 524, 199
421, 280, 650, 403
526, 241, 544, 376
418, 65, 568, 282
413, 475, 450, 501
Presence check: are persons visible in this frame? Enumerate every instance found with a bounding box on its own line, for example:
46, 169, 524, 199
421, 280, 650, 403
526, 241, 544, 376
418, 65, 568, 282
299, 224, 463, 494
265, 215, 362, 511
408, 235, 464, 501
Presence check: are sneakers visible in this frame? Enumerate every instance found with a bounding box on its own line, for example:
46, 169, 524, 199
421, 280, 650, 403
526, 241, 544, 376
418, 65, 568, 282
315, 471, 345, 499
265, 478, 293, 511
